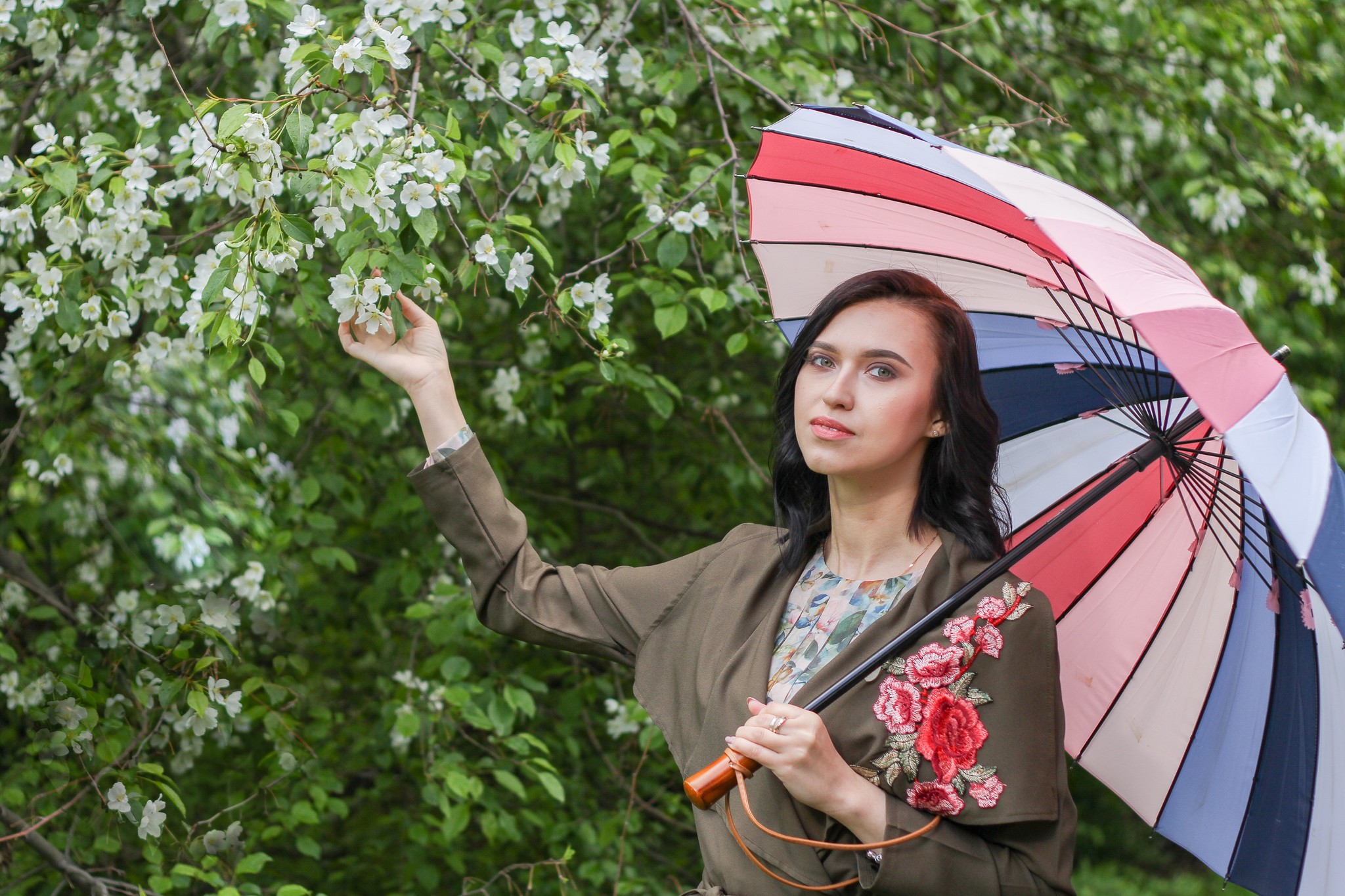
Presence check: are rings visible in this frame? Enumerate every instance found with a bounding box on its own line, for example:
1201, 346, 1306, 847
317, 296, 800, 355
770, 715, 788, 737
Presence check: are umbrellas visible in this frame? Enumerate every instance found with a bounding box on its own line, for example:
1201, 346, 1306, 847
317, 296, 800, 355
681, 101, 1345, 896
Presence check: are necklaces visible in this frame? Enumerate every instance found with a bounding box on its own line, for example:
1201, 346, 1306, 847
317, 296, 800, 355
898, 531, 941, 579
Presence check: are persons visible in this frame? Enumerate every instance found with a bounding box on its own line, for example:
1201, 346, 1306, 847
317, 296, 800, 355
337, 269, 1080, 894
